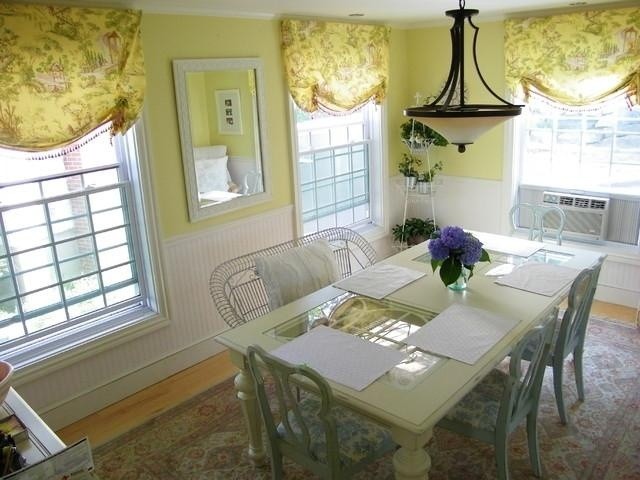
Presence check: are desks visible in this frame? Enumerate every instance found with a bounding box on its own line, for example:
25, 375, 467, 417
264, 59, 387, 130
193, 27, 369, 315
215, 229, 607, 480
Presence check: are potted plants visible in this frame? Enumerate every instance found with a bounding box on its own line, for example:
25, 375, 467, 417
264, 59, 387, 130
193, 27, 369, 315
392, 118, 443, 248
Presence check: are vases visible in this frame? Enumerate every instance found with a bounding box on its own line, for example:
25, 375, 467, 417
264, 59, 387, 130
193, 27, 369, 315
447, 267, 467, 291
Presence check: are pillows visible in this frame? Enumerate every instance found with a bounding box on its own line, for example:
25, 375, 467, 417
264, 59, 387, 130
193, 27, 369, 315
254, 238, 343, 312
195, 155, 229, 193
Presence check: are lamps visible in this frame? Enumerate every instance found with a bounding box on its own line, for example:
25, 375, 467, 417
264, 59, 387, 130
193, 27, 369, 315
403, 0, 526, 153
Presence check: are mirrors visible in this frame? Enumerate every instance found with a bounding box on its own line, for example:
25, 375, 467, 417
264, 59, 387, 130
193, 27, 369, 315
171, 57, 274, 225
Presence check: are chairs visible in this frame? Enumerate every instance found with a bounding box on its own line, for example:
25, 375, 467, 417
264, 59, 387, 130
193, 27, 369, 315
245, 171, 262, 195
192, 145, 227, 162
507, 201, 567, 246
246, 343, 401, 480
436, 327, 549, 480
508, 263, 601, 425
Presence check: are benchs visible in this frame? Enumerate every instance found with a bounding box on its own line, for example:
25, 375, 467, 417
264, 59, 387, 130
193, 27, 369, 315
209, 226, 378, 328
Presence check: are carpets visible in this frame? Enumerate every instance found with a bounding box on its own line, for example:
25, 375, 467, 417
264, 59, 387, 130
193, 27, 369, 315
78, 308, 640, 480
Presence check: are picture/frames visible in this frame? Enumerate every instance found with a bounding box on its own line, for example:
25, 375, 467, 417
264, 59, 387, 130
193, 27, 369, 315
214, 88, 243, 135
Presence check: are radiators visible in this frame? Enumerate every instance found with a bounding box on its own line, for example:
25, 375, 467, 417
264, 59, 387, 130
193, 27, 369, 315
542, 192, 611, 244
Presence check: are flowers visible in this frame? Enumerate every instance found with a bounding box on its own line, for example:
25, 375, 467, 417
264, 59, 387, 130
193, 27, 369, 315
428, 225, 492, 287
